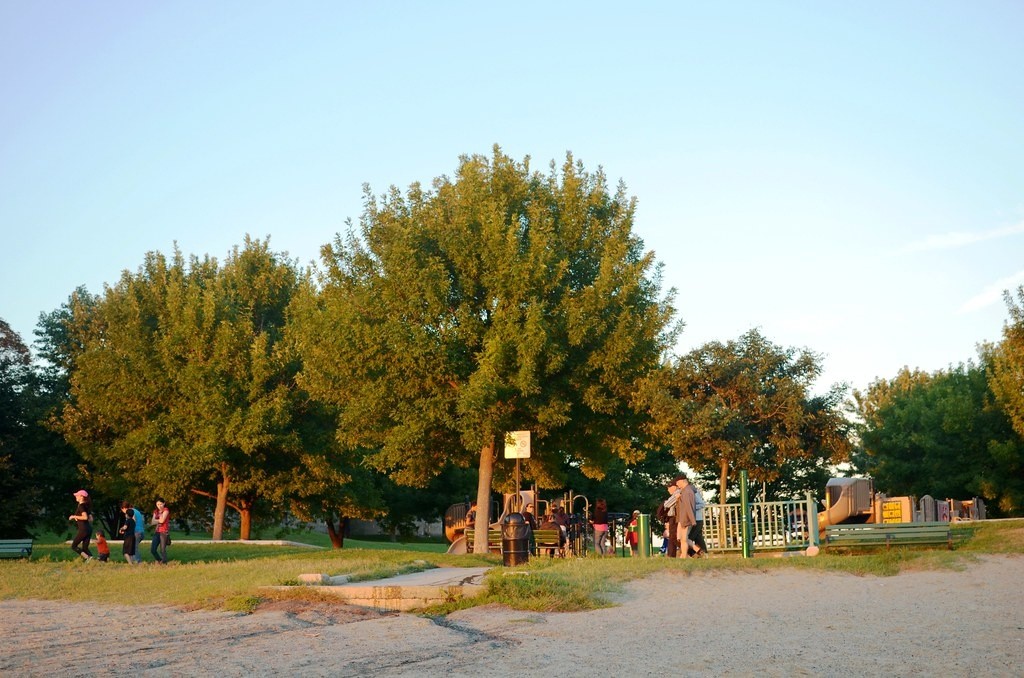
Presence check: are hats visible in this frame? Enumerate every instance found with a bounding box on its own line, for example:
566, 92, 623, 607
674, 475, 686, 482
74, 490, 88, 497
666, 479, 677, 487
634, 510, 640, 514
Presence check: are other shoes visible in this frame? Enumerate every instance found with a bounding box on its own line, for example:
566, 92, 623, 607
84, 556, 93, 565
696, 549, 705, 558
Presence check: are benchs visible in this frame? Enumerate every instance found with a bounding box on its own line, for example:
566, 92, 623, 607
703, 522, 788, 546
0, 539, 34, 560
825, 521, 954, 553
465, 528, 565, 557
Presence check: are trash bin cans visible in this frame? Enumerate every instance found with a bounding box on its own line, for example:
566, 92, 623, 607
500, 512, 531, 567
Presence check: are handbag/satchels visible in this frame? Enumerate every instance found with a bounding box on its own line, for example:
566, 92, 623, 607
165, 535, 171, 545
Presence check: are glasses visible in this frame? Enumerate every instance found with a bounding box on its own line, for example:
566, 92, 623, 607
528, 506, 535, 508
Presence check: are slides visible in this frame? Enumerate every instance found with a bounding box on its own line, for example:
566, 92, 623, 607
817, 490, 848, 530
446, 505, 524, 554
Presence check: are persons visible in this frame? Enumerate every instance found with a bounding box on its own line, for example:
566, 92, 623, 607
150, 497, 169, 565
589, 497, 609, 555
119, 503, 145, 564
523, 502, 571, 559
464, 501, 477, 553
658, 474, 708, 558
69, 490, 110, 563
626, 509, 643, 556
